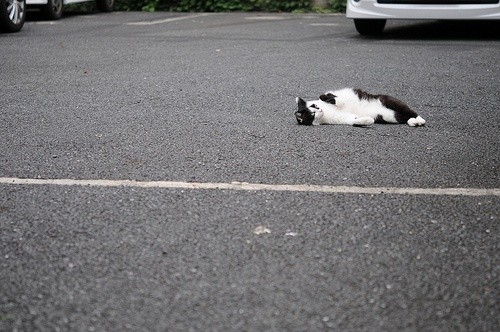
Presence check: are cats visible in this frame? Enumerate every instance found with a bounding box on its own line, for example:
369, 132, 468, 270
293, 87, 426, 128
